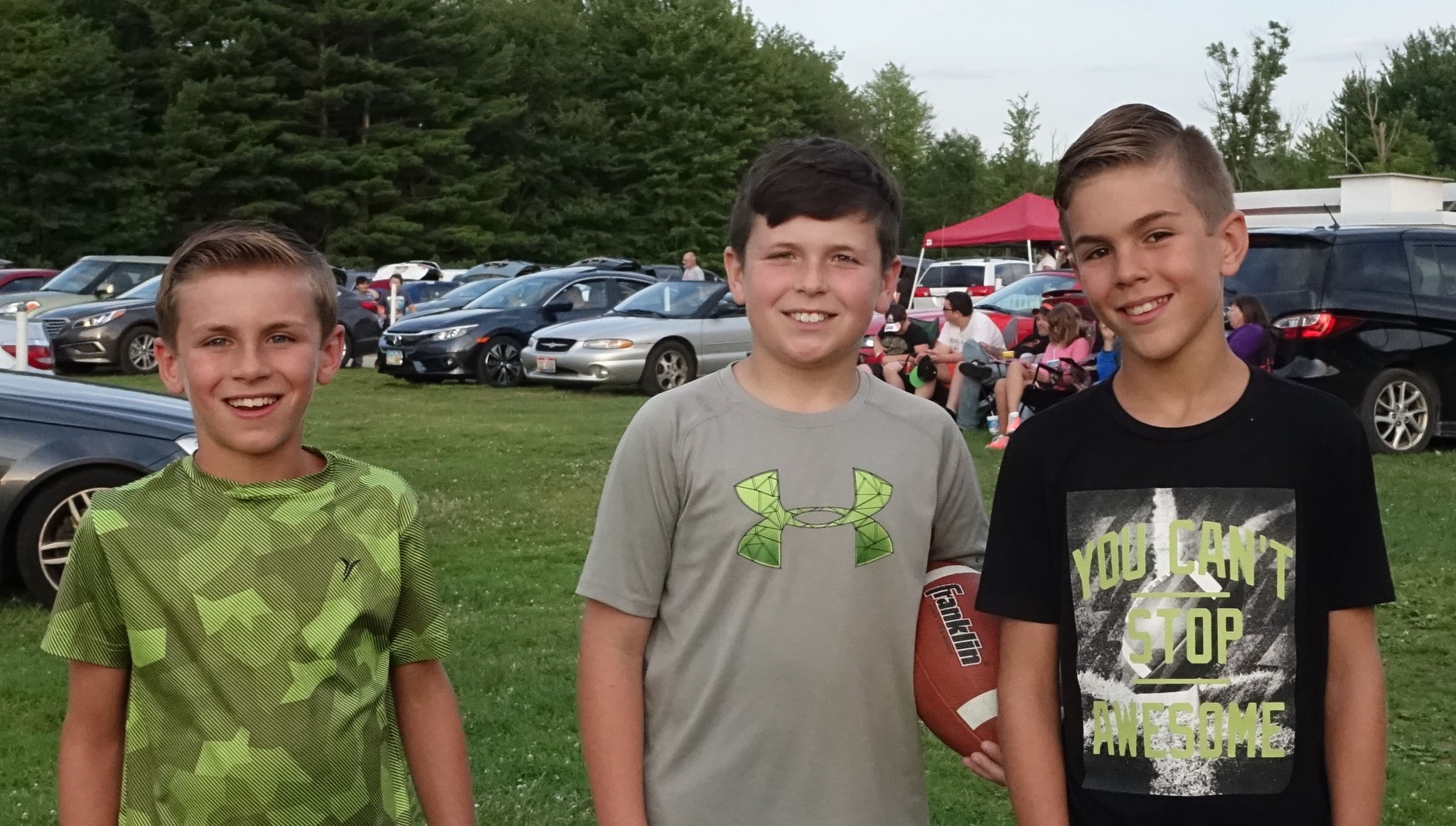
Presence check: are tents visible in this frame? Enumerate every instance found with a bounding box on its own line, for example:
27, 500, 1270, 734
908, 192, 1063, 309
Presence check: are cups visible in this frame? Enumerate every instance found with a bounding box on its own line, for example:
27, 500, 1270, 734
1048, 359, 1059, 383
1002, 351, 1014, 358
987, 415, 999, 434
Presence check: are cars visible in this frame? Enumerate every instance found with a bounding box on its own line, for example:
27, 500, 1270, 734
0, 371, 199, 614
893, 255, 939, 309
371, 264, 682, 389
30, 269, 386, 378
859, 266, 1098, 378
518, 278, 754, 398
0, 252, 181, 377
327, 255, 757, 399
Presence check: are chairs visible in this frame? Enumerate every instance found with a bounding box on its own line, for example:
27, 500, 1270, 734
978, 362, 1078, 431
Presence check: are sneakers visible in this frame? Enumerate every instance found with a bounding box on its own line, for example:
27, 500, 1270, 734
985, 434, 1009, 451
1005, 415, 1023, 436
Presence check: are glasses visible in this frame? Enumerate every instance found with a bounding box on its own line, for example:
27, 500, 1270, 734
942, 306, 956, 311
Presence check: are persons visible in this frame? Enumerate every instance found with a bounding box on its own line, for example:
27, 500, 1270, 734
42, 218, 476, 826
682, 252, 705, 280
895, 267, 916, 309
379, 273, 416, 323
579, 135, 1005, 826
956, 304, 1054, 431
984, 302, 1090, 449
975, 104, 1397, 826
1095, 320, 1117, 379
1035, 240, 1057, 271
1227, 295, 1270, 365
873, 303, 929, 392
915, 291, 1007, 415
342, 276, 370, 368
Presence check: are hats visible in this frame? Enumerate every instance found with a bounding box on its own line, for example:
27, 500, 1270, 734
884, 304, 907, 332
1031, 302, 1057, 316
910, 354, 938, 388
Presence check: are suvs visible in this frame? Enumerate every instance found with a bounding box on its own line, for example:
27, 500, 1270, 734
912, 256, 1052, 314
1219, 221, 1456, 458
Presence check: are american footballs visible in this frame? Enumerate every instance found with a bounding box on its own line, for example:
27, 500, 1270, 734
914, 559, 1002, 758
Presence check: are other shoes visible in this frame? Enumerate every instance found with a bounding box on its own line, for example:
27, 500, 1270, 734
958, 361, 993, 380
